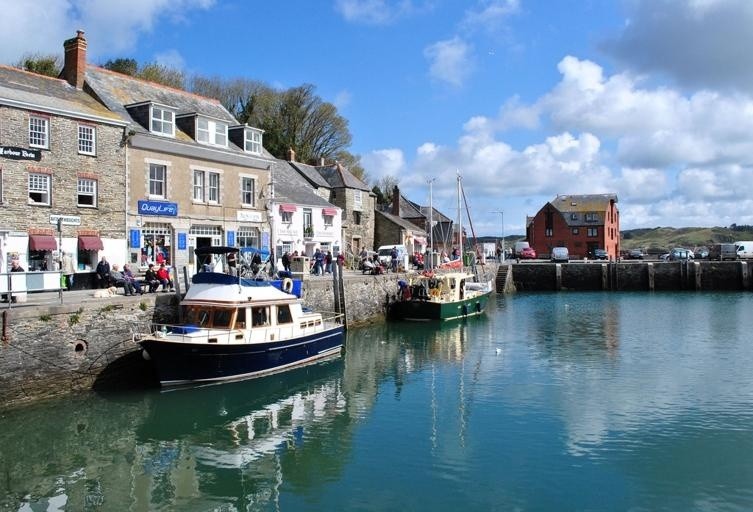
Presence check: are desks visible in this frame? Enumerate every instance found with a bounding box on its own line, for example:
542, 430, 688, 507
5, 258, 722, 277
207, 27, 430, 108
487, 210, 505, 262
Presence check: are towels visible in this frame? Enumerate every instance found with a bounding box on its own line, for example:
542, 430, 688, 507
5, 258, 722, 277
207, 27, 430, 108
428, 253, 440, 267
291, 256, 311, 277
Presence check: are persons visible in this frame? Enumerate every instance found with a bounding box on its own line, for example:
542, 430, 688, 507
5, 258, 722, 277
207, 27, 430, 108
397, 277, 412, 301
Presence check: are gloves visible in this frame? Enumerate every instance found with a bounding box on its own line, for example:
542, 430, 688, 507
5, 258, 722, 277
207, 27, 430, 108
395, 174, 494, 323
134, 242, 348, 389
400, 315, 495, 453
138, 361, 348, 512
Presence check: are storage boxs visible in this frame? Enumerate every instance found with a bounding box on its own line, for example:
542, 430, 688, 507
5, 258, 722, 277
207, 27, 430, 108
363, 261, 392, 274
110, 272, 149, 294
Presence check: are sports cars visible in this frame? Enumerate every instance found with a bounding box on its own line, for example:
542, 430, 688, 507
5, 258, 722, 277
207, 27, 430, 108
360, 257, 373, 274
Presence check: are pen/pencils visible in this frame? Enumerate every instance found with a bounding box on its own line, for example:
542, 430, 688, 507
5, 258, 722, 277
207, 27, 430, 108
377, 245, 406, 267
551, 247, 569, 262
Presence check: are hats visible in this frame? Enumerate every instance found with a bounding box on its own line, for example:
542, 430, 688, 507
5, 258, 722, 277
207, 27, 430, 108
658, 241, 752, 260
519, 248, 536, 259
628, 249, 644, 260
592, 248, 608, 260
